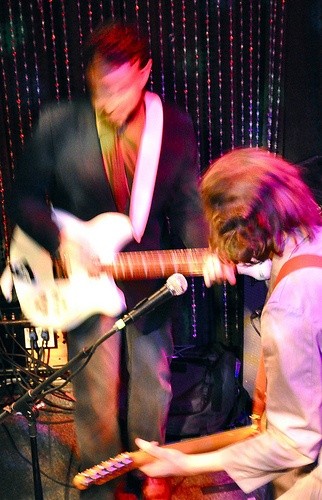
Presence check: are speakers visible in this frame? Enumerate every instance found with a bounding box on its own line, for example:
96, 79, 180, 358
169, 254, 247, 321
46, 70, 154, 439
240, 274, 268, 398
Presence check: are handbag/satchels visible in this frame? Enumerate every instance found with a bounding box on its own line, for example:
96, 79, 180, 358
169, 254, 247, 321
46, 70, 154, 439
169, 469, 271, 499
163, 337, 249, 443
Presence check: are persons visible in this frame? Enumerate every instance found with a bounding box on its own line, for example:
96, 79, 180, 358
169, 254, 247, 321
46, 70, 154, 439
135, 148, 322, 500
16, 26, 235, 500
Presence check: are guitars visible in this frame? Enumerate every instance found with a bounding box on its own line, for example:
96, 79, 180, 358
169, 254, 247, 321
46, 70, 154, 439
9, 208, 272, 334
73, 410, 267, 490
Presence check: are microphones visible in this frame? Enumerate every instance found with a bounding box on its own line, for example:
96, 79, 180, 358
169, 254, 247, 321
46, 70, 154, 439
250, 306, 264, 318
114, 273, 188, 331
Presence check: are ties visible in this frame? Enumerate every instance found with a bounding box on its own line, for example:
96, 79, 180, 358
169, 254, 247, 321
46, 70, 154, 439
109, 132, 132, 215
